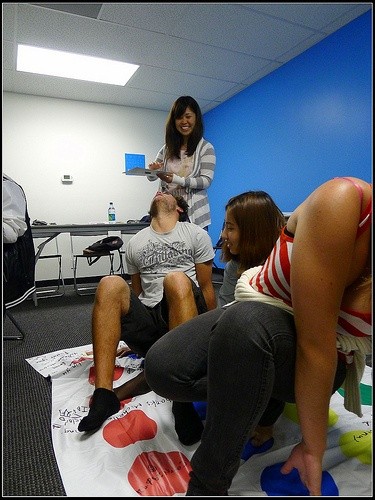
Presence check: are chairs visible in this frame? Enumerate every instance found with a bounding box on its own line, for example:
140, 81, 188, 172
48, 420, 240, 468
3, 231, 61, 340
213, 220, 226, 278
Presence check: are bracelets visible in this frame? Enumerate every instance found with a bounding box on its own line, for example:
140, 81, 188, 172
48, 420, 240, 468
165, 175, 169, 181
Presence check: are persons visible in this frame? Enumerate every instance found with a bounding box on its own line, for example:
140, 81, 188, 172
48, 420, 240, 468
145, 176, 372, 496
216, 191, 283, 308
3, 173, 36, 309
78, 193, 216, 430
147, 96, 215, 231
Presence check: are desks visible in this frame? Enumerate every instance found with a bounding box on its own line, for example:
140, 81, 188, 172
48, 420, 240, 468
30, 223, 181, 306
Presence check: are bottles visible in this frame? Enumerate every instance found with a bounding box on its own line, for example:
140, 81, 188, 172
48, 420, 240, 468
108, 202, 115, 224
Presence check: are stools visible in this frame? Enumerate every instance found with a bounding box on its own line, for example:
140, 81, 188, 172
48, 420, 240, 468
73, 236, 128, 295
